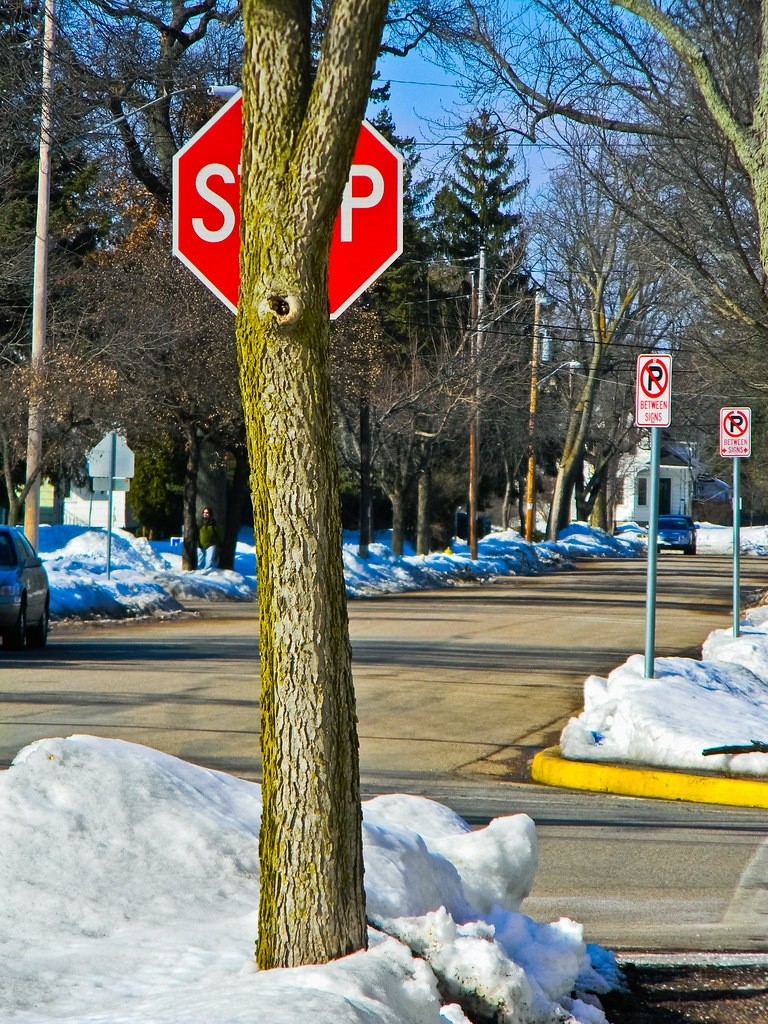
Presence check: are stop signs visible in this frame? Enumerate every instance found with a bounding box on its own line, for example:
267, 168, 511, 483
172, 89, 405, 321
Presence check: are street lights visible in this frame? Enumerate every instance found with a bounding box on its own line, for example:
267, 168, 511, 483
469, 297, 550, 554
25, 83, 240, 549
525, 360, 580, 539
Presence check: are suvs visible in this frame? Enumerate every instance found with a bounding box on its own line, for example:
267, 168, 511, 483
645, 514, 700, 554
0, 526, 51, 655
